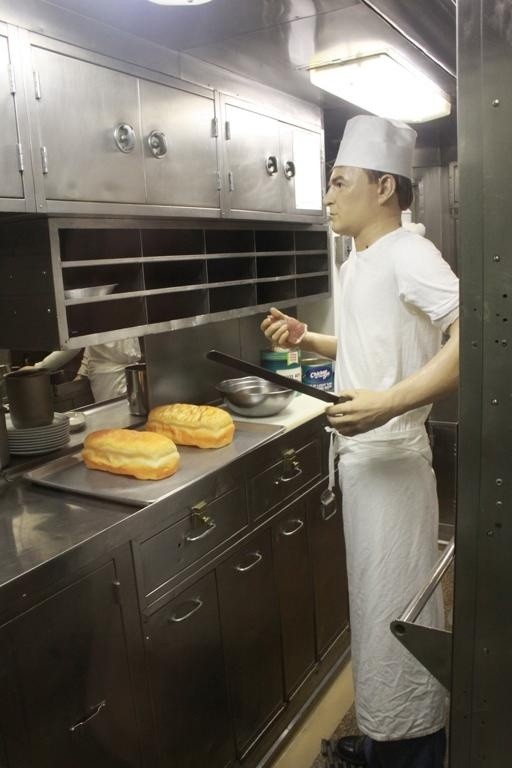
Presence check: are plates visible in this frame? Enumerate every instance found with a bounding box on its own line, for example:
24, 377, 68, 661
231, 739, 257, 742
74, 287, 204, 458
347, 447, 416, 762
62, 282, 118, 301
6, 411, 70, 454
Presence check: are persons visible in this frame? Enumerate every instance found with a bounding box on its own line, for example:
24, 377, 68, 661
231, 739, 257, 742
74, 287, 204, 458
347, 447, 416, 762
260, 114, 460, 768
34, 337, 141, 404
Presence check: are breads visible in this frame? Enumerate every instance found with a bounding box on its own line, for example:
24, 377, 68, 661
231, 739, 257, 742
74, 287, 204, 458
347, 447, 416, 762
147, 403, 235, 448
81, 429, 180, 481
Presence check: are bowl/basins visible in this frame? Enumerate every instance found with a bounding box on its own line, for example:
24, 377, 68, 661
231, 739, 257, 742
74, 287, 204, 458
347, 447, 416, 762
214, 375, 293, 417
59, 410, 87, 431
126, 364, 146, 417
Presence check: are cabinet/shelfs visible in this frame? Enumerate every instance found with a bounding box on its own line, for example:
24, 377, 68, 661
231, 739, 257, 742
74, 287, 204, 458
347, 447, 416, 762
1, 21, 223, 217
223, 90, 323, 216
2, 436, 351, 767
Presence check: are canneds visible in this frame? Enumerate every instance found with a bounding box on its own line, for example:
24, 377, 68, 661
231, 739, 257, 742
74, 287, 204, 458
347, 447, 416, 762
302, 358, 334, 391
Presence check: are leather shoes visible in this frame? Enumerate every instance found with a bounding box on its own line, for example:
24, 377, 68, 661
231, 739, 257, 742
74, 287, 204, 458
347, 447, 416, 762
331, 735, 369, 765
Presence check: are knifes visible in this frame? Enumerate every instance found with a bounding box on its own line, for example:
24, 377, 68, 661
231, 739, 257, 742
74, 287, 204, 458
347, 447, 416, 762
207, 351, 352, 404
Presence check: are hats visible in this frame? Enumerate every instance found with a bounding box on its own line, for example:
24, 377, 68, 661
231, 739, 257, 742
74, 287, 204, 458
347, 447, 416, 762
330, 114, 420, 183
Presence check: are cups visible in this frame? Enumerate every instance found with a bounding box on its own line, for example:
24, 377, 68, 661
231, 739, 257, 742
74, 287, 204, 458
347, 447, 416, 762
5, 369, 53, 427
